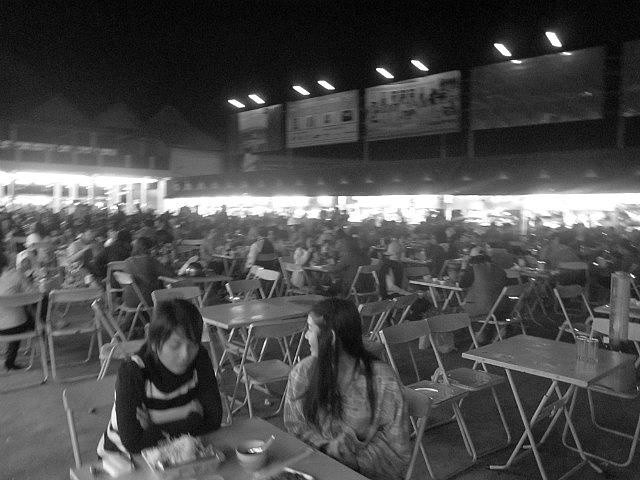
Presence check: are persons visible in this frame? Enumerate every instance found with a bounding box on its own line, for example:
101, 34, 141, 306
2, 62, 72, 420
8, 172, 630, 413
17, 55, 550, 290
2, 193, 640, 375
93, 297, 224, 464
282, 297, 413, 479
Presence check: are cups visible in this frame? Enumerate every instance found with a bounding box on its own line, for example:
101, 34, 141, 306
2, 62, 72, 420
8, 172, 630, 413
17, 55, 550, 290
537, 262, 546, 270
577, 334, 599, 363
420, 273, 464, 287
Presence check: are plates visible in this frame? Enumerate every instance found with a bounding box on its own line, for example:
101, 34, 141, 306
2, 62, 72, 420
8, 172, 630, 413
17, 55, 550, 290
260, 466, 317, 480
140, 446, 228, 478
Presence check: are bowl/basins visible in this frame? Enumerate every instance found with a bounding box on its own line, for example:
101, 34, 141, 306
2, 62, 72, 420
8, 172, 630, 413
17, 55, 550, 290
235, 440, 268, 471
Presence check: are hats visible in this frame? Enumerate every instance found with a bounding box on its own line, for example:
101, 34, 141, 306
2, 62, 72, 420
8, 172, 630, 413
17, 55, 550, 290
22, 232, 43, 248
470, 245, 485, 257
